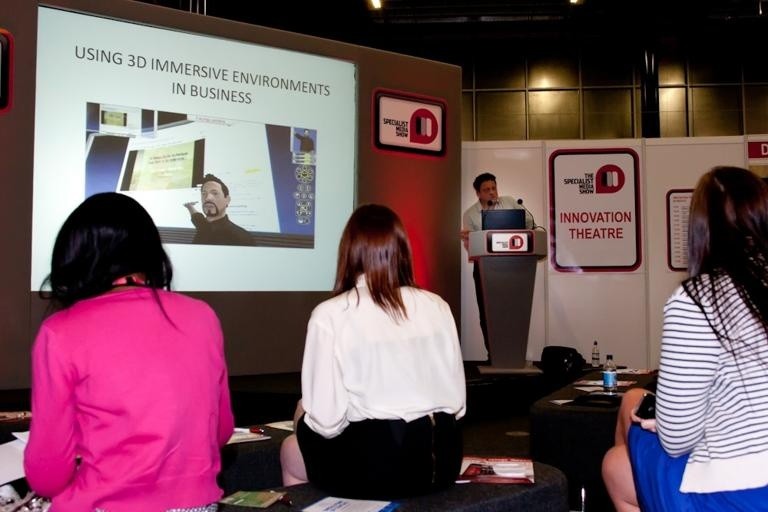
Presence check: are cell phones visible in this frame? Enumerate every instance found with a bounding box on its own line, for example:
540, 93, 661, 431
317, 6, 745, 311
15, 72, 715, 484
590, 391, 617, 396
634, 392, 655, 419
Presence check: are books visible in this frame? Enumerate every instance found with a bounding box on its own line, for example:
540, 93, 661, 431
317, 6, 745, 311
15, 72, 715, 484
226, 407, 297, 446
0, 431, 29, 486
221, 483, 396, 512
456, 455, 535, 484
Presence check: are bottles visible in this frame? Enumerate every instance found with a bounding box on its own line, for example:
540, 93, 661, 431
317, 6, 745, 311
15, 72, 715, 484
604, 354, 617, 394
591, 341, 601, 368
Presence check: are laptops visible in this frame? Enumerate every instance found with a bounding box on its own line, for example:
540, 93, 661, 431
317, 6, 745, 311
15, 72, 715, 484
482, 210, 525, 230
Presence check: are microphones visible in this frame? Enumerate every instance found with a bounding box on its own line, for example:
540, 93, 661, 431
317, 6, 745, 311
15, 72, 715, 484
482, 200, 492, 230
518, 199, 534, 230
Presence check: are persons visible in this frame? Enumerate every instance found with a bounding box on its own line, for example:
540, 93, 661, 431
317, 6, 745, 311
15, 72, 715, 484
599, 167, 768, 512
23, 194, 234, 512
459, 158, 537, 363
295, 129, 314, 153
186, 174, 246, 247
280, 203, 468, 493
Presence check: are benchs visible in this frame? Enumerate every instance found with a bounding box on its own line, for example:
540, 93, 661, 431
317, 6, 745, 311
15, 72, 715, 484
215, 457, 566, 512
529, 367, 657, 510
